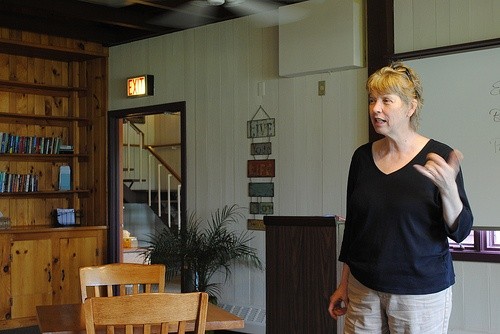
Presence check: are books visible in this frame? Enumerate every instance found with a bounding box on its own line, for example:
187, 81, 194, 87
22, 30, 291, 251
58, 166, 71, 190
52, 208, 76, 225
0, 131, 74, 155
0, 171, 37, 192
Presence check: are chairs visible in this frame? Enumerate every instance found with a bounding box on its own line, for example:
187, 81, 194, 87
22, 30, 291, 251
78, 261, 209, 334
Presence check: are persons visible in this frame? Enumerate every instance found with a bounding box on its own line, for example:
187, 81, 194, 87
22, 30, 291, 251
328, 64, 473, 334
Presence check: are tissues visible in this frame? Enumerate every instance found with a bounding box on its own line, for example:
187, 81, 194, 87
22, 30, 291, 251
123, 230, 138, 248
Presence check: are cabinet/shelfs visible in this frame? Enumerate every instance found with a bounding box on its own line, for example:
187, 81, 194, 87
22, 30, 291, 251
0, 27, 110, 332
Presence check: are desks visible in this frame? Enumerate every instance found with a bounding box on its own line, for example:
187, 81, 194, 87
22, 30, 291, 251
262, 215, 345, 334
37, 302, 244, 334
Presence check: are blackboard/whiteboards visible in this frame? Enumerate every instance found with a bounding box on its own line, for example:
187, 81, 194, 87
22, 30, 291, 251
392, 45, 500, 230
